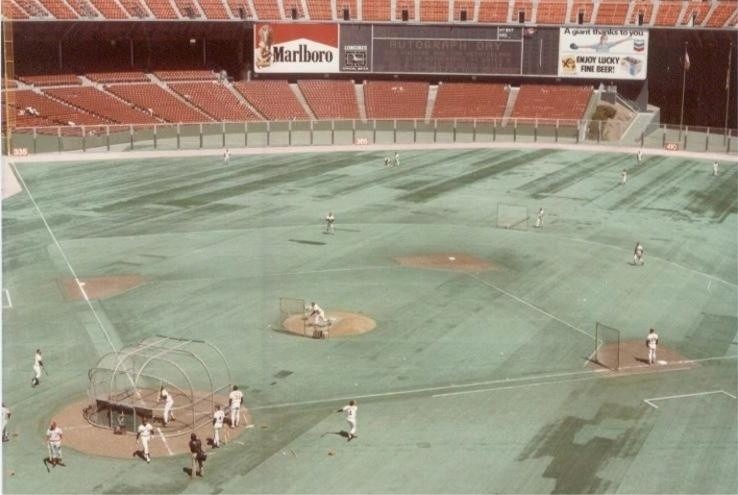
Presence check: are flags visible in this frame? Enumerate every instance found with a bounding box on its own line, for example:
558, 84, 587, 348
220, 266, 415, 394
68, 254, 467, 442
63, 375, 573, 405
684, 50, 691, 70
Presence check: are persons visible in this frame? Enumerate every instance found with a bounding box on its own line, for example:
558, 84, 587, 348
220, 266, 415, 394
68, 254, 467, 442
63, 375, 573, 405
188, 432, 206, 479
31, 348, 44, 386
334, 398, 358, 440
325, 211, 335, 233
634, 242, 645, 266
305, 301, 330, 324
212, 403, 225, 449
160, 384, 176, 427
636, 149, 643, 166
228, 384, 244, 429
383, 156, 392, 167
45, 421, 65, 466
2, 400, 13, 443
645, 327, 659, 365
713, 159, 719, 175
223, 148, 231, 166
394, 152, 399, 166
570, 33, 633, 54
135, 416, 155, 463
620, 167, 628, 184
533, 207, 545, 227
